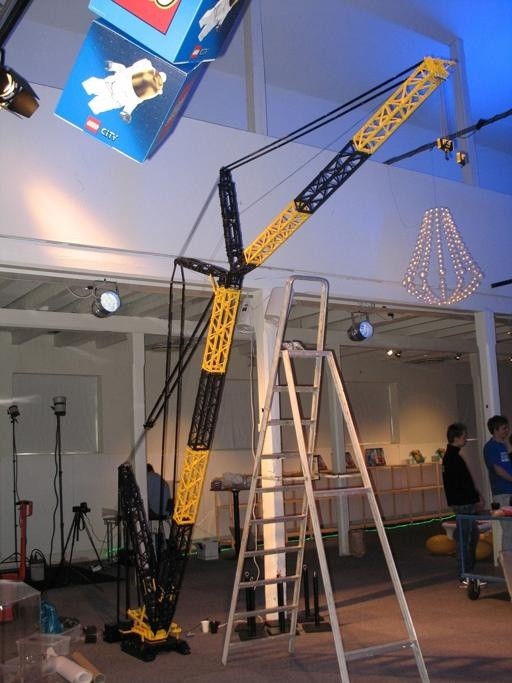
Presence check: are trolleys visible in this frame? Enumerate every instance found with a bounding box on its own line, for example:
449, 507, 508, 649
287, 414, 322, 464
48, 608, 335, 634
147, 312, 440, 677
454, 512, 512, 601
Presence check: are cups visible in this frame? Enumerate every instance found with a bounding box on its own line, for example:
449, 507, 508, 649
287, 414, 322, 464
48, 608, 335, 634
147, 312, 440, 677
210, 621, 219, 633
201, 620, 210, 633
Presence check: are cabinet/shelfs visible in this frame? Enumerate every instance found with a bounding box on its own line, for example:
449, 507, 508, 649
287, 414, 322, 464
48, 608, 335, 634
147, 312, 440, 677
210, 461, 455, 553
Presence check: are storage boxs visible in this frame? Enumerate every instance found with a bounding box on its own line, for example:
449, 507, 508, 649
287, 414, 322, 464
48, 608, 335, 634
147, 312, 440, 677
16, 633, 71, 683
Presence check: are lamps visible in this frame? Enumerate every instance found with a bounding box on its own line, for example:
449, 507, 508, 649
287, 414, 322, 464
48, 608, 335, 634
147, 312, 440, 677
0, 64, 40, 118
91, 279, 123, 318
347, 311, 373, 342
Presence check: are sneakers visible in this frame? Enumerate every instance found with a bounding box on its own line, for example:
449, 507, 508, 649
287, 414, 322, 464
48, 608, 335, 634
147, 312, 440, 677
460, 579, 487, 588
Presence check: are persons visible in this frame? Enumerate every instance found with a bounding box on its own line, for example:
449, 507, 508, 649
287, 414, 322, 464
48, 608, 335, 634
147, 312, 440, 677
146, 460, 172, 518
440, 420, 489, 589
481, 413, 511, 566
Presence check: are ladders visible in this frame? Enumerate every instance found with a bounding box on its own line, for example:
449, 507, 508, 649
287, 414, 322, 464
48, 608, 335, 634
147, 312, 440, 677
219, 274, 430, 682
